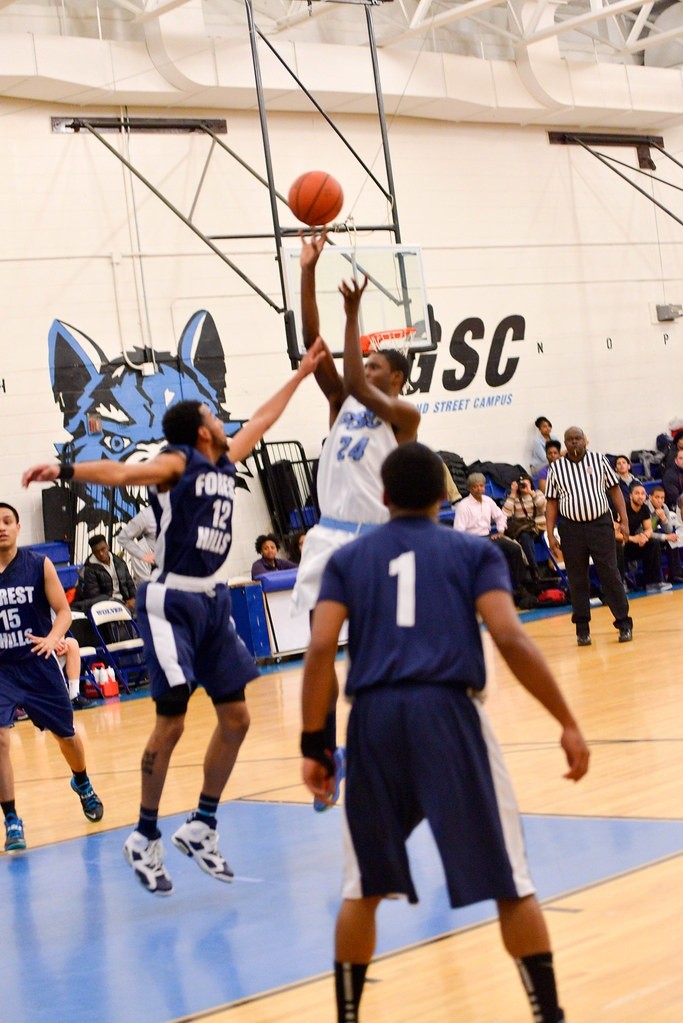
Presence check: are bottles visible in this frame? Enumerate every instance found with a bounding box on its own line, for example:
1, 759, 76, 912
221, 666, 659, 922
85, 665, 115, 685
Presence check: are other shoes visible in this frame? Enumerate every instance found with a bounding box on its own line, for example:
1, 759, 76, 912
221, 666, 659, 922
577, 634, 591, 646
619, 629, 631, 642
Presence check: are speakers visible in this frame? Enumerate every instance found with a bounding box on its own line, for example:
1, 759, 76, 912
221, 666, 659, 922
42, 487, 76, 541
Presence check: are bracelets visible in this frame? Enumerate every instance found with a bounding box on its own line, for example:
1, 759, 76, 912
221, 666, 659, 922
57, 464, 74, 479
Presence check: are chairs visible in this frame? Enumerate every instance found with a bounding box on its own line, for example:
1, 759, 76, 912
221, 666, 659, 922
67, 599, 147, 702
543, 527, 594, 592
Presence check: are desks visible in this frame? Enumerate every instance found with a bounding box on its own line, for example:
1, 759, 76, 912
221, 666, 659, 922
230, 569, 348, 663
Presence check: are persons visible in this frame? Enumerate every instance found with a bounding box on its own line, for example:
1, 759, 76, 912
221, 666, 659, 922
531, 417, 558, 477
434, 428, 683, 607
84, 535, 137, 624
289, 530, 344, 652
292, 223, 421, 812
545, 426, 634, 646
0, 502, 104, 850
301, 440, 590, 1023
251, 533, 299, 661
22, 335, 327, 898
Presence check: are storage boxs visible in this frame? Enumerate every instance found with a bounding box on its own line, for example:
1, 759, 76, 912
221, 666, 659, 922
57, 565, 85, 588
24, 543, 70, 564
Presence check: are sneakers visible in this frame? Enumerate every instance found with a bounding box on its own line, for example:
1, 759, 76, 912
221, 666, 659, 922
4, 818, 26, 850
70, 775, 103, 822
171, 820, 233, 883
122, 831, 173, 896
645, 582, 672, 593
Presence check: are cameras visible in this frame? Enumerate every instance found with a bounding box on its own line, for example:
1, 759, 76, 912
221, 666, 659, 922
516, 479, 526, 488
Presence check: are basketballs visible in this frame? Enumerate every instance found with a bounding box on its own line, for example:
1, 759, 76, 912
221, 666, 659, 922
288, 169, 344, 226
